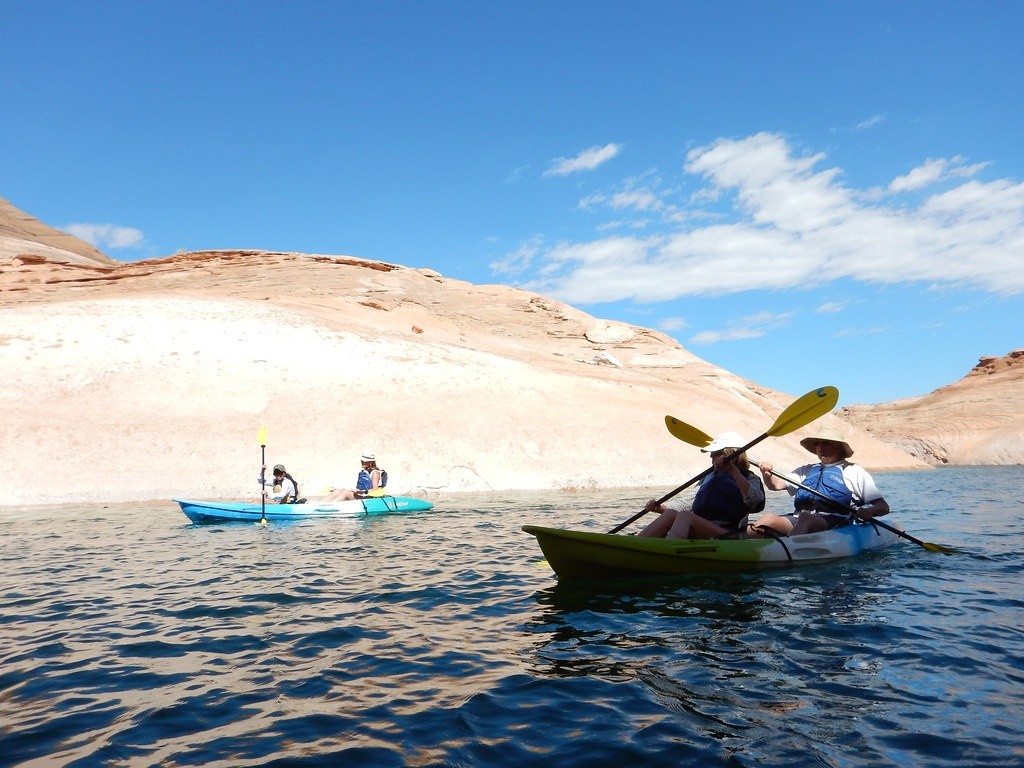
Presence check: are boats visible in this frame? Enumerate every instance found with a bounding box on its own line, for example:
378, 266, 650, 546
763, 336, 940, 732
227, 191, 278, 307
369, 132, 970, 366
520, 517, 905, 587
173, 495, 434, 527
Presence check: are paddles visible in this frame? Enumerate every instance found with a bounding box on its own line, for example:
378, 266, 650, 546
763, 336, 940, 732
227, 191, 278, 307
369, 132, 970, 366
533, 386, 840, 568
257, 428, 270, 526
664, 414, 953, 555
321, 487, 384, 497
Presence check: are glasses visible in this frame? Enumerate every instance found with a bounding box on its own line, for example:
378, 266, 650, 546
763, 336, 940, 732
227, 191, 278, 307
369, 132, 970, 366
274, 470, 283, 476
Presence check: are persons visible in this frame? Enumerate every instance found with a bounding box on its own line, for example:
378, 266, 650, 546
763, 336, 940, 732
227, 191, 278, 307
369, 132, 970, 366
747, 425, 890, 539
637, 430, 765, 542
257, 464, 298, 504
320, 451, 384, 502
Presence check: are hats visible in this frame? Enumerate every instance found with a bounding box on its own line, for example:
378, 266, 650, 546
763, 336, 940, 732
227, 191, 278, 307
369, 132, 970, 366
700, 432, 746, 453
800, 424, 854, 458
272, 464, 286, 475
361, 453, 375, 462
